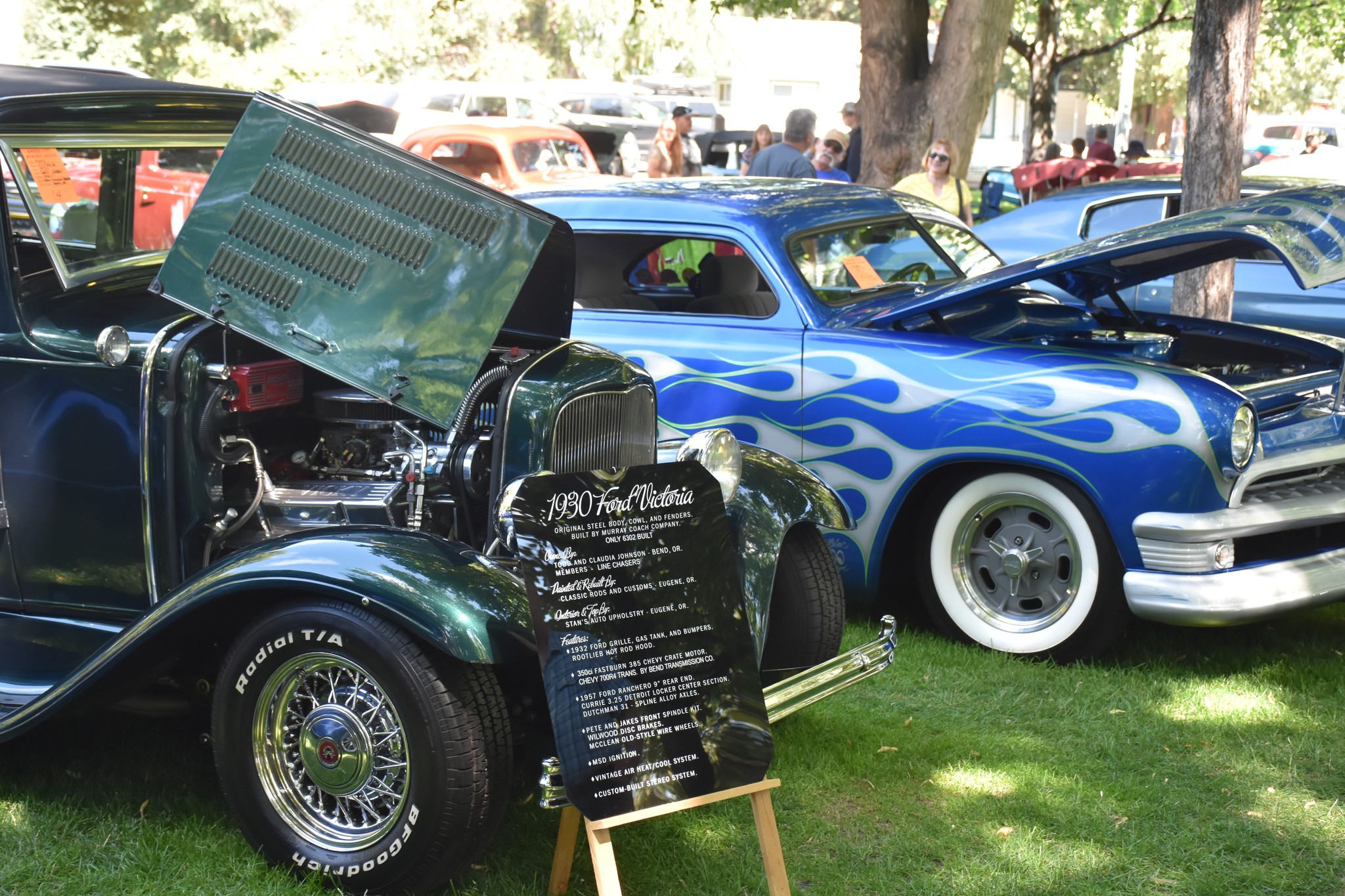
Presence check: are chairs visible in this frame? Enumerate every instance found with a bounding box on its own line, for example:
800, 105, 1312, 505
687, 256, 777, 316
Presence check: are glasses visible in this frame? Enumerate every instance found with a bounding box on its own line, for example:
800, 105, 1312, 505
822, 140, 842, 152
929, 151, 947, 162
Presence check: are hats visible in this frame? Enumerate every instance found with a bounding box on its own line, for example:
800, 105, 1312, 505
672, 107, 694, 119
823, 129, 850, 152
1120, 140, 1152, 158
838, 102, 856, 114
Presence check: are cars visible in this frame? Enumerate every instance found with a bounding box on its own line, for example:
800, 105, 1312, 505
1240, 112, 1345, 194
3, 146, 227, 250
0, 61, 902, 895
845, 179, 1345, 337
278, 80, 821, 195
501, 175, 1345, 663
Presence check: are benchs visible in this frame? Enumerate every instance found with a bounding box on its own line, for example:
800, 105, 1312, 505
573, 294, 659, 310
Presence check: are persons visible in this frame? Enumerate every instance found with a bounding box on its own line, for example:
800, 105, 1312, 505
746, 109, 853, 290
803, 136, 820, 160
647, 106, 703, 180
1300, 134, 1321, 155
739, 124, 774, 176
1120, 141, 1151, 177
1086, 125, 1119, 184
1029, 121, 1053, 203
839, 101, 862, 182
1043, 137, 1086, 193
888, 138, 974, 239
